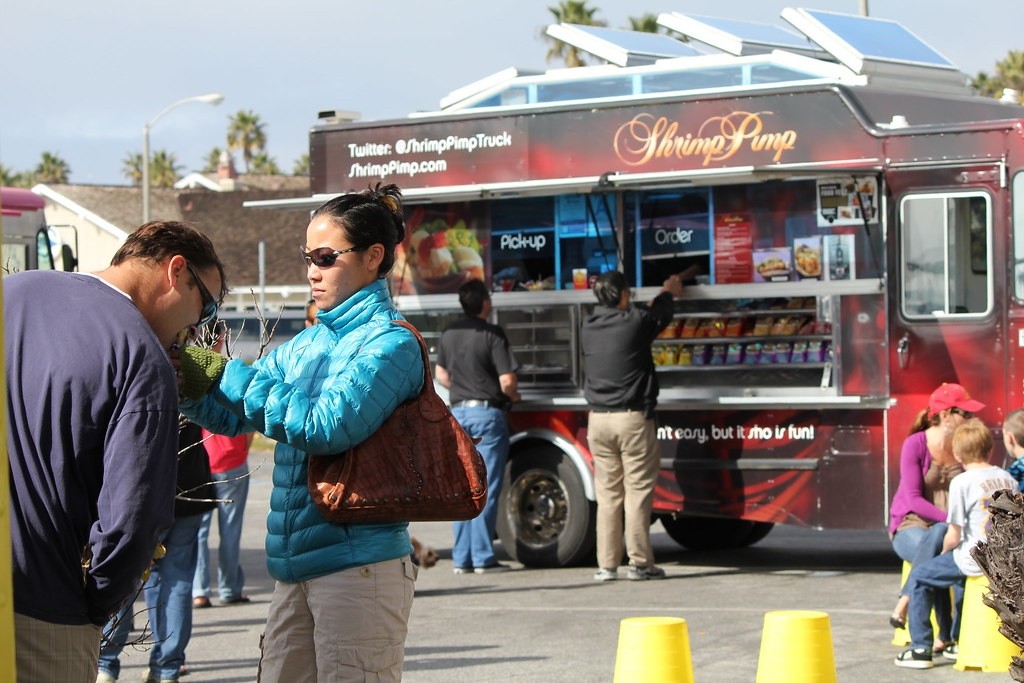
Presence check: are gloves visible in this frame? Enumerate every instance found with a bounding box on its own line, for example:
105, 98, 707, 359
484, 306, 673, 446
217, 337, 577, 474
171, 344, 229, 399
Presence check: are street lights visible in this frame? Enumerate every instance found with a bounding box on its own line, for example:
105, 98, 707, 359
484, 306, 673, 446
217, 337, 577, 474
136, 91, 229, 225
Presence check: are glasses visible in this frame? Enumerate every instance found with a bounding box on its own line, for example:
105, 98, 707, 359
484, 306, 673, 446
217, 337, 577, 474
300, 245, 358, 269
950, 408, 973, 419
186, 264, 218, 328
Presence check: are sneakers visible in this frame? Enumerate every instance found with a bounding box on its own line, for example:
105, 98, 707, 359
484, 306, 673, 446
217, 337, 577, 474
595, 567, 618, 581
629, 565, 667, 579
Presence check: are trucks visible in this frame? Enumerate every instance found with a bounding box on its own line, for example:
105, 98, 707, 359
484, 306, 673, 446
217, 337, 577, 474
239, 4, 1024, 572
0, 185, 82, 276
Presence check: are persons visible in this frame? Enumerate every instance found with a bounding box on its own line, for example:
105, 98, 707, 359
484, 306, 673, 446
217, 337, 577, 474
192, 322, 255, 607
171, 184, 413, 683
894, 418, 1020, 668
96, 416, 217, 683
2, 222, 228, 683
584, 270, 685, 579
1002, 409, 1024, 494
435, 279, 522, 573
889, 384, 986, 653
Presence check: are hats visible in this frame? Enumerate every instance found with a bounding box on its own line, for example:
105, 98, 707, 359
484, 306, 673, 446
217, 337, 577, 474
929, 382, 986, 412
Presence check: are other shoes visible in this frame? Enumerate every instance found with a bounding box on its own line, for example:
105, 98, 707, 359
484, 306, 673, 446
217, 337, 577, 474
143, 667, 178, 683
942, 645, 959, 661
895, 648, 933, 669
454, 566, 472, 575
891, 615, 908, 630
192, 597, 210, 608
474, 562, 512, 573
97, 672, 114, 683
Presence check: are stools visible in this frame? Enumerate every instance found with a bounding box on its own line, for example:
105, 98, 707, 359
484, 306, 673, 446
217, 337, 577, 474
612, 616, 694, 683
892, 558, 953, 652
954, 575, 1022, 675
756, 608, 837, 683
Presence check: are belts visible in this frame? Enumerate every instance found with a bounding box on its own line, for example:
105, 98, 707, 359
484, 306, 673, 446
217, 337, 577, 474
591, 406, 645, 412
452, 398, 503, 409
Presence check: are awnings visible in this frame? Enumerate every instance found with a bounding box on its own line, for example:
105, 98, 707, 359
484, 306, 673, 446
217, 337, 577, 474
244, 163, 885, 287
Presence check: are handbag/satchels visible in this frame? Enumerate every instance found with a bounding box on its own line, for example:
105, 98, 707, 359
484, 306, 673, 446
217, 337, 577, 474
308, 321, 487, 526
